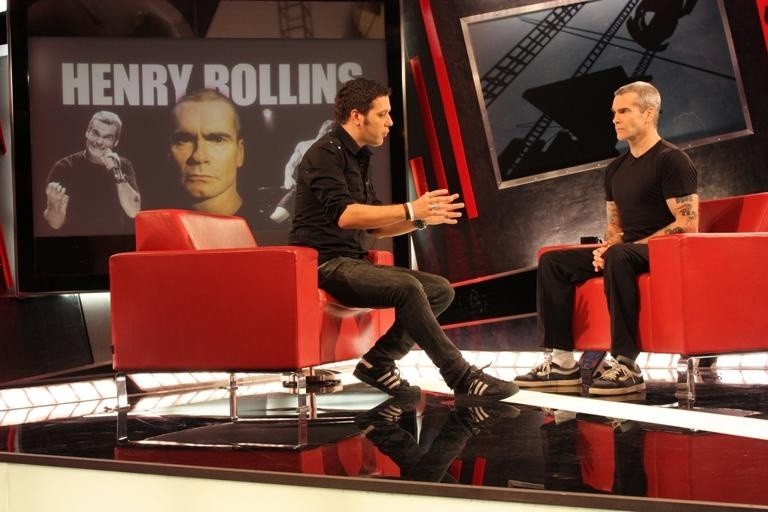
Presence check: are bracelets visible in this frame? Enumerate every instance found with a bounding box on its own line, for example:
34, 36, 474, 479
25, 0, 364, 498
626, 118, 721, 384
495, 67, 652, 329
402, 200, 413, 222
411, 221, 426, 230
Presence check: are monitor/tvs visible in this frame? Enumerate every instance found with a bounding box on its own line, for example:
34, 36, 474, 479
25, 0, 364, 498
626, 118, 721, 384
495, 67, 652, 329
7, 0, 409, 296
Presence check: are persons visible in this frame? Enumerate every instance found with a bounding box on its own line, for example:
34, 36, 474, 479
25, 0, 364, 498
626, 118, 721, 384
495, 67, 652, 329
352, 393, 522, 483
510, 79, 700, 393
42, 108, 147, 235
160, 88, 293, 246
286, 76, 522, 405
537, 407, 651, 498
269, 116, 337, 227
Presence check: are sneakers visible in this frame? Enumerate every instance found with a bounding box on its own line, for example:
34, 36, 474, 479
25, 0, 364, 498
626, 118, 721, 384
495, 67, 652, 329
454, 362, 519, 401
353, 358, 421, 396
455, 400, 520, 436
512, 352, 583, 387
588, 359, 647, 395
355, 396, 419, 434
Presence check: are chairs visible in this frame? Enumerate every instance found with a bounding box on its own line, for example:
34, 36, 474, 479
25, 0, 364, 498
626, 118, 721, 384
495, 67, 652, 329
536, 190, 768, 411
107, 207, 396, 451
